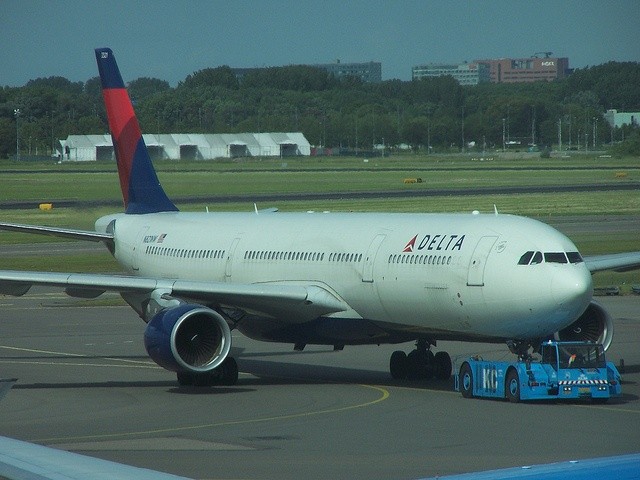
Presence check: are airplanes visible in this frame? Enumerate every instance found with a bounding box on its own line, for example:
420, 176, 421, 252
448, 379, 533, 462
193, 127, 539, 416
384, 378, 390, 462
0, 42, 639, 386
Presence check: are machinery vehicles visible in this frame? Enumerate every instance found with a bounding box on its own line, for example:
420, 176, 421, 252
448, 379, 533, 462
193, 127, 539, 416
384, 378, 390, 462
455, 340, 625, 405
